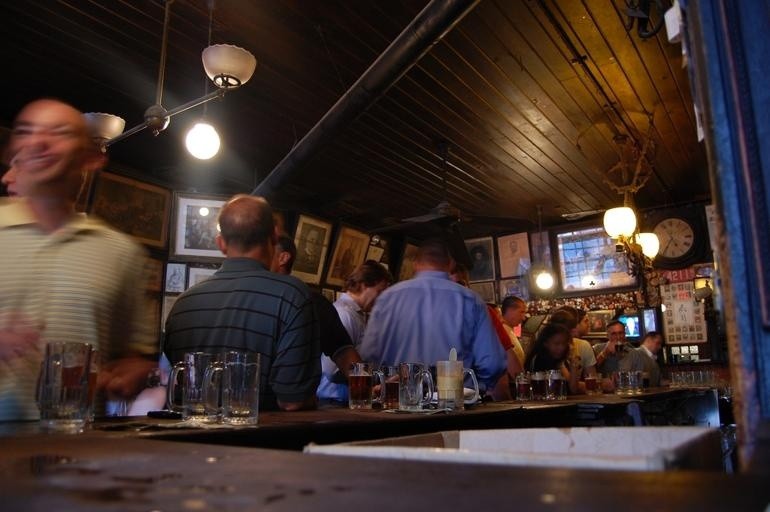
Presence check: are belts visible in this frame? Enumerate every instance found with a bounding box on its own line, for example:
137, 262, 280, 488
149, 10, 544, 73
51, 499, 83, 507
320, 398, 349, 407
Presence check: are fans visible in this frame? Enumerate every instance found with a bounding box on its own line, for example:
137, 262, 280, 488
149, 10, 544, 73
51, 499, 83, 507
401, 145, 537, 233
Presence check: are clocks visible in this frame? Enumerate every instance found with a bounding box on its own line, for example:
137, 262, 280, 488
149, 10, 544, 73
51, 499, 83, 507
640, 206, 705, 270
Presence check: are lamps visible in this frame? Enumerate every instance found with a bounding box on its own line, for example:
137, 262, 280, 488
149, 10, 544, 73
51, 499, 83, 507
693, 275, 713, 299
495, 228, 533, 280
535, 206, 553, 290
576, 105, 659, 270
497, 276, 529, 304
469, 282, 496, 306
84, 0, 258, 159
185, 0, 221, 161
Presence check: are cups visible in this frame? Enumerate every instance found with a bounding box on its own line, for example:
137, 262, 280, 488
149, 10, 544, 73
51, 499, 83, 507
54, 347, 104, 424
168, 349, 221, 418
585, 373, 599, 394
347, 362, 386, 409
202, 350, 261, 424
616, 367, 627, 393
438, 359, 479, 412
33, 338, 92, 430
670, 371, 719, 387
532, 370, 548, 399
515, 371, 530, 400
550, 370, 567, 401
398, 358, 432, 412
380, 363, 410, 409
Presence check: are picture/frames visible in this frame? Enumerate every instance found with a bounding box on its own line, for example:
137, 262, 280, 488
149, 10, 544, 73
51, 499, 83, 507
323, 222, 372, 292
395, 243, 420, 280
82, 167, 233, 332
463, 235, 497, 284
285, 213, 333, 288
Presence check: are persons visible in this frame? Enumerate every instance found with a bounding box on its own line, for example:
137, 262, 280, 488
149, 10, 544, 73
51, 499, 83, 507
0, 97, 167, 422
485, 294, 663, 401
3, 148, 25, 200
164, 193, 324, 409
450, 264, 528, 402
267, 235, 362, 381
467, 243, 494, 280
294, 225, 326, 275
316, 258, 393, 404
503, 239, 527, 273
364, 244, 510, 401
